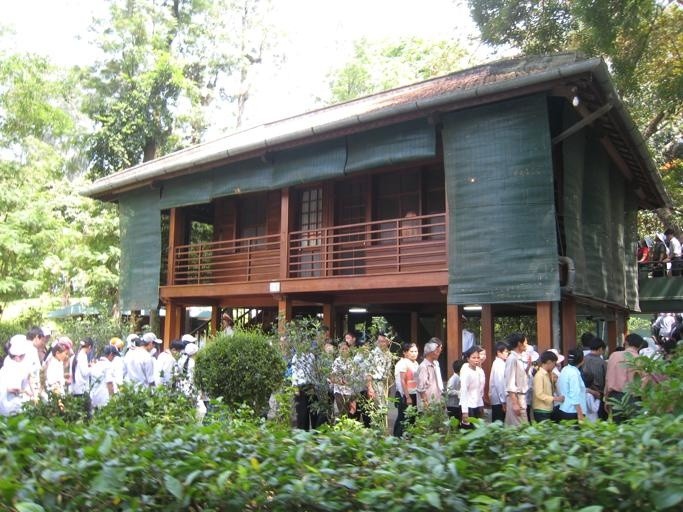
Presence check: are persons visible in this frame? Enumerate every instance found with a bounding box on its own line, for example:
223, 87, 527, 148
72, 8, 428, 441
0, 312, 682, 418
638, 229, 683, 277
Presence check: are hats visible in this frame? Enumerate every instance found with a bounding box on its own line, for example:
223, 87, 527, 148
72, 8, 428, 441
644, 237, 651, 248
222, 313, 234, 327
522, 345, 539, 362
424, 343, 439, 356
41, 327, 51, 337
110, 337, 124, 348
58, 337, 74, 354
182, 334, 197, 342
547, 349, 565, 363
143, 332, 163, 344
126, 334, 139, 340
9, 335, 32, 356
185, 343, 198, 355
656, 232, 667, 242
104, 345, 120, 356
